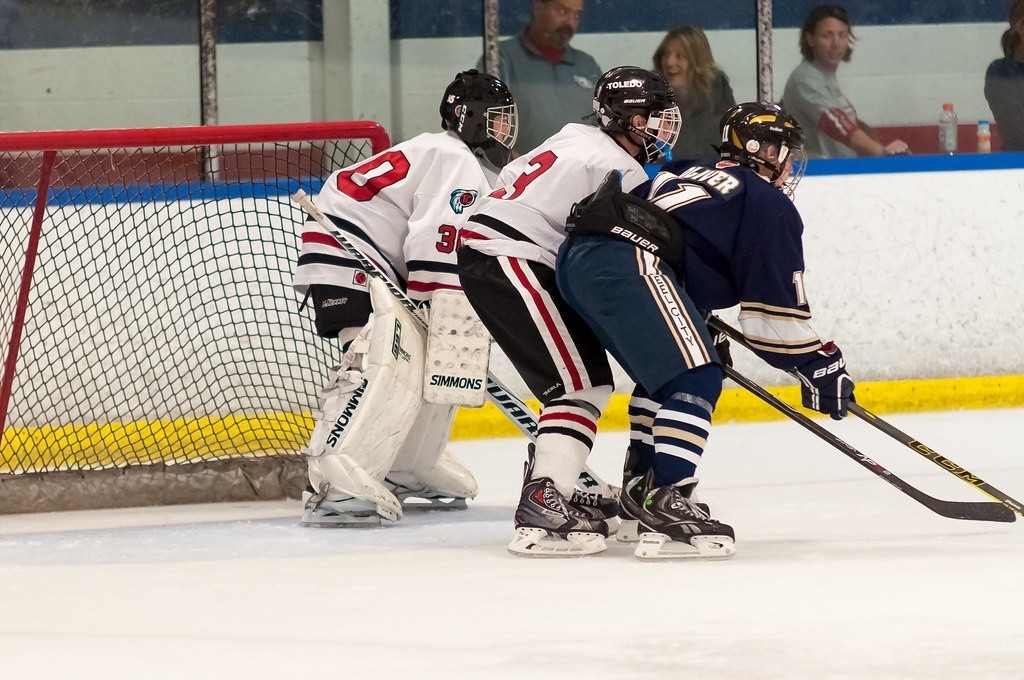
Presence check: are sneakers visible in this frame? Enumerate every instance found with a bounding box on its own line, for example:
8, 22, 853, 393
635, 477, 735, 560
616, 469, 710, 542
518, 443, 620, 536
508, 461, 609, 556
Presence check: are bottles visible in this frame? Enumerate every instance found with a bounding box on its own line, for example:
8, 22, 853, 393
977, 120, 992, 153
939, 104, 958, 154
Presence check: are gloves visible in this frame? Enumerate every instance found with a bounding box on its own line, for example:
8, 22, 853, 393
794, 341, 855, 420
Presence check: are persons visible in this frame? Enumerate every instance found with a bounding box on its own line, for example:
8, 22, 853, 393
292, 66, 857, 560
475, 0, 604, 155
650, 24, 737, 162
984, 25, 1024, 151
779, 5, 913, 156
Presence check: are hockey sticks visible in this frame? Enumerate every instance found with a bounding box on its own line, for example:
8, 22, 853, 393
722, 359, 1016, 521
290, 188, 622, 502
699, 307, 1024, 515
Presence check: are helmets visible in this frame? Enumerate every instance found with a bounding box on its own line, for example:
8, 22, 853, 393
720, 101, 809, 194
593, 66, 677, 164
440, 69, 514, 169
